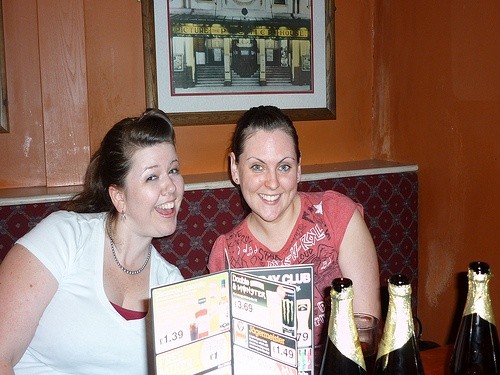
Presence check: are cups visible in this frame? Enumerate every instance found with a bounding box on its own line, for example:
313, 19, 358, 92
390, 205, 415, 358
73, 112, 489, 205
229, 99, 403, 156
353, 313, 378, 357
414, 315, 422, 353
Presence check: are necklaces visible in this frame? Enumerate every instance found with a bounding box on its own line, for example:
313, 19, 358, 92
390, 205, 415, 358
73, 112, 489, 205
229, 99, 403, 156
109, 213, 152, 275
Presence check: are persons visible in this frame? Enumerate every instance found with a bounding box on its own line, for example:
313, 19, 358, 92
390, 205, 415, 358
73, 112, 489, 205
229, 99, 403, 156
0, 108, 183, 375
205, 105, 380, 375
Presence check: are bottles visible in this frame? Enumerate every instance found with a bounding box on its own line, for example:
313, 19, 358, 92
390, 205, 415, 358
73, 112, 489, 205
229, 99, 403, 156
318, 277, 368, 375
447, 261, 500, 375
372, 274, 425, 375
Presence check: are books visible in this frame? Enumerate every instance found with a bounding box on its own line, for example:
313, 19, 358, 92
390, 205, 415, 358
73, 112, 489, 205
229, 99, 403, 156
150, 248, 314, 375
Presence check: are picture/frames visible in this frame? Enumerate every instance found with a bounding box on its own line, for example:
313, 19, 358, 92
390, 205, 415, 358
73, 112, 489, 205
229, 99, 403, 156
140, 0, 337, 126
